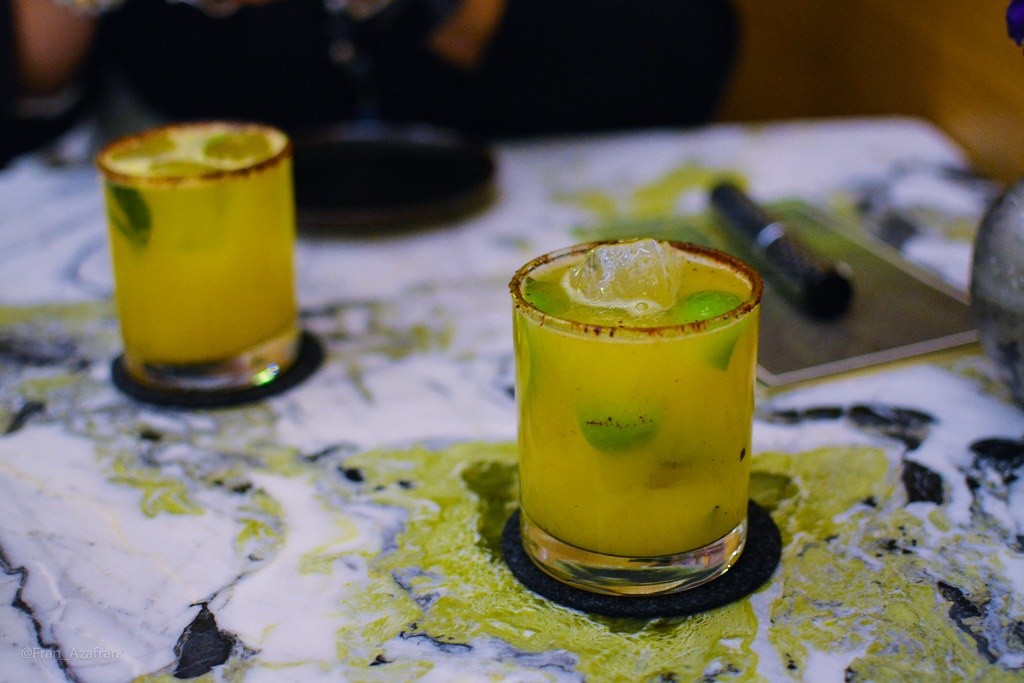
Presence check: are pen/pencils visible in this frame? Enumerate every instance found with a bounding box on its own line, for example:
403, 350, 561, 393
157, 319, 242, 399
709, 177, 859, 323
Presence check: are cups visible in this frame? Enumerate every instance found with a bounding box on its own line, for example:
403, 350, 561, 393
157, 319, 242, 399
509, 238, 765, 598
92, 119, 302, 393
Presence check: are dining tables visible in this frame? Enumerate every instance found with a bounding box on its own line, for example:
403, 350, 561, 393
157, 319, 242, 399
0, 114, 1023, 683
